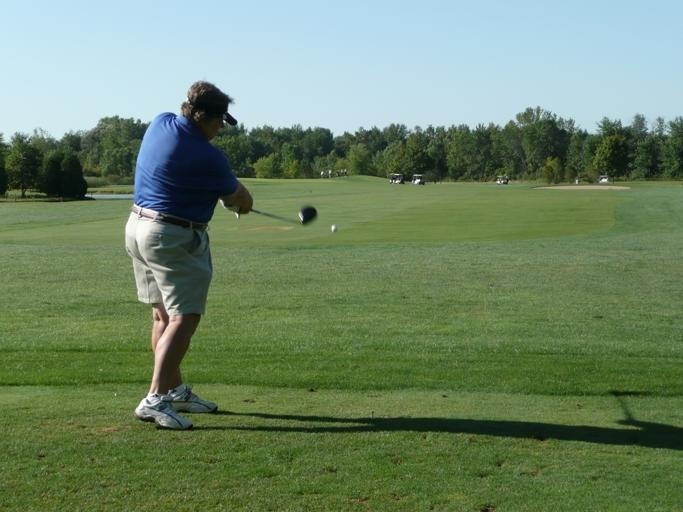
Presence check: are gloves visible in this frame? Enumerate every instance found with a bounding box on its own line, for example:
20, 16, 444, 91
220, 183, 253, 218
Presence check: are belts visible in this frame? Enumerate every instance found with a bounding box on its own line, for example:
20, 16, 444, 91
132, 203, 207, 231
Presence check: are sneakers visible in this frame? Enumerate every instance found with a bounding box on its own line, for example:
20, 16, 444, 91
134, 387, 218, 430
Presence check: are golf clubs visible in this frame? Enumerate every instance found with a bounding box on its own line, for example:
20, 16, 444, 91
249, 206, 317, 224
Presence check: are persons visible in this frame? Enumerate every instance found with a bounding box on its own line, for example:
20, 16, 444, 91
124, 82, 254, 430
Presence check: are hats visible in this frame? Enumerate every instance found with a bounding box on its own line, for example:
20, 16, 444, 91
186, 95, 237, 127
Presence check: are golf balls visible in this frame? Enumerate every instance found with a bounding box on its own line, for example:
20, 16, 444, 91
331, 225, 337, 232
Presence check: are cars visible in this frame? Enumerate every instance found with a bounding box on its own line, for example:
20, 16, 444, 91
599, 175, 610, 183
